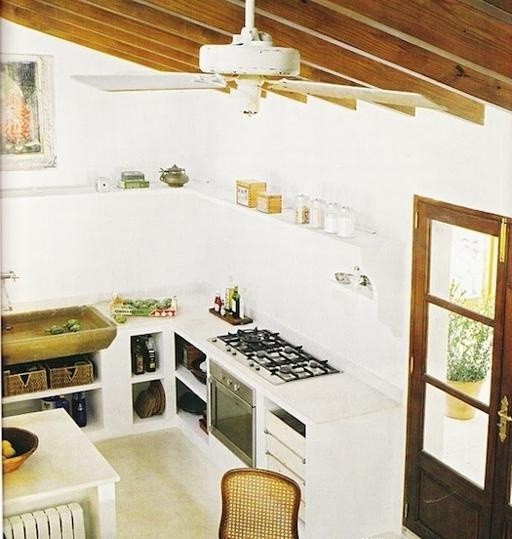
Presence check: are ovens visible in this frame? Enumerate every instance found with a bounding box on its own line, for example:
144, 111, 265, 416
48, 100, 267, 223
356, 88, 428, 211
206, 359, 255, 469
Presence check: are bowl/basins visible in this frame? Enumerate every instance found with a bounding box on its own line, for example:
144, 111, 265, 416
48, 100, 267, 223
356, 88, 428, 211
2, 426, 39, 475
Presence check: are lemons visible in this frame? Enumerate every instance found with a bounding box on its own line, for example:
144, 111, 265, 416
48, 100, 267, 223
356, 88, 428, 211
2, 440, 15, 460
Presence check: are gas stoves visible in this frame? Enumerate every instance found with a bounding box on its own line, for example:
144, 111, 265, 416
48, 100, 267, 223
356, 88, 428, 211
206, 326, 340, 386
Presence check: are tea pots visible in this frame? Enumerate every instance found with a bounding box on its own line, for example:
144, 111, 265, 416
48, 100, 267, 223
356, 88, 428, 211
160, 164, 189, 187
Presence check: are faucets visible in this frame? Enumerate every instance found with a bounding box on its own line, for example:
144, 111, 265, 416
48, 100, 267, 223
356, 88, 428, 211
1, 272, 19, 283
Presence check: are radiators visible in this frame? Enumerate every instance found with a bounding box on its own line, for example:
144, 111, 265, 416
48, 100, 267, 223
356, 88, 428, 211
2, 502, 86, 539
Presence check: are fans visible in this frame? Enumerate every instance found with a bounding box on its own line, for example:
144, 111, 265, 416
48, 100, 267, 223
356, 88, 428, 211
67, 0, 448, 117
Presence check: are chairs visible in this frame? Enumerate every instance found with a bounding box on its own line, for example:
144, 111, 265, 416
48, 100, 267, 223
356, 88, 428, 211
217, 468, 301, 539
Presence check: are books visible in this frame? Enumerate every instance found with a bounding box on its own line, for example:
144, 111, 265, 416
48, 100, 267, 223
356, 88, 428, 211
118, 179, 150, 190
121, 171, 145, 179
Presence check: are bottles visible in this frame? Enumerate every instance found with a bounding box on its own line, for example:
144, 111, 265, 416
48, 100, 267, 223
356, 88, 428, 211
133, 336, 145, 375
41, 391, 86, 427
146, 334, 158, 372
215, 284, 240, 319
295, 192, 356, 241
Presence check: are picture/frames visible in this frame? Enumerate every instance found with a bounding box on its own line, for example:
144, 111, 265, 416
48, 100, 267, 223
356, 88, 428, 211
0, 52, 58, 170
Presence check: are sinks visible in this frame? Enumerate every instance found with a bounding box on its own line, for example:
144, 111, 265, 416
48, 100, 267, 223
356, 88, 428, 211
1, 305, 116, 366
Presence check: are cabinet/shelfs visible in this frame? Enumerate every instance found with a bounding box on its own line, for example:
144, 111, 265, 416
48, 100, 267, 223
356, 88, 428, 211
259, 392, 404, 539
1, 322, 208, 457
